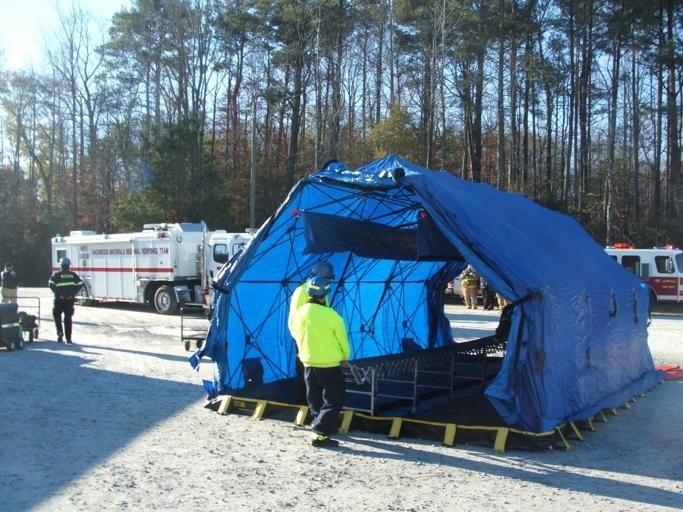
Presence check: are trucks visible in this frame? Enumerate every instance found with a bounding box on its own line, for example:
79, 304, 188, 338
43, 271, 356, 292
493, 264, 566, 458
51, 219, 260, 315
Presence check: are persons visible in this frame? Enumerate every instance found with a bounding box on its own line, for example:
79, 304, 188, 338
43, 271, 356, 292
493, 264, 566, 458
287, 260, 335, 405
0, 263, 17, 302
48, 256, 84, 343
289, 276, 350, 447
460, 266, 479, 309
480, 276, 492, 310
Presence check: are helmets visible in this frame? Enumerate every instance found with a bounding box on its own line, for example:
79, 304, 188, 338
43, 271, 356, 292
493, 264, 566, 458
307, 276, 331, 296
312, 261, 334, 279
59, 257, 71, 265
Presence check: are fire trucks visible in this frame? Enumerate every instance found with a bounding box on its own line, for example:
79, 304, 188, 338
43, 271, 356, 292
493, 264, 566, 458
603, 243, 683, 310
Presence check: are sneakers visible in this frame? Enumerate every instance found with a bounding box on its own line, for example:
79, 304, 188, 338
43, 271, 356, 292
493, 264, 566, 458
313, 434, 340, 447
58, 334, 72, 343
